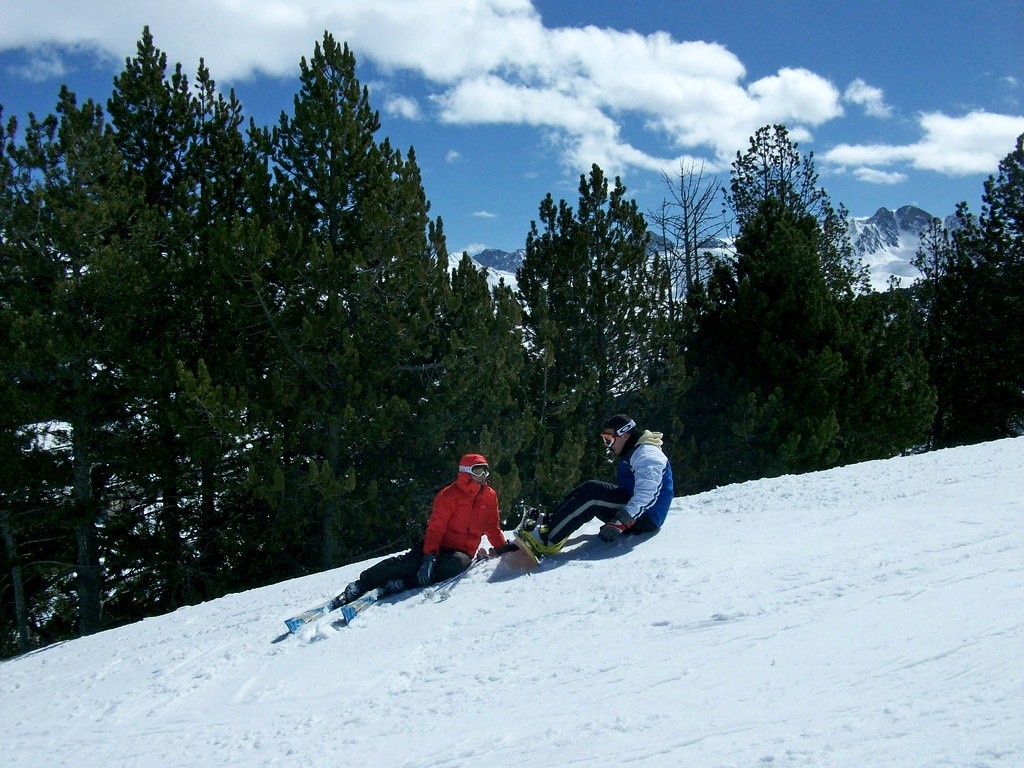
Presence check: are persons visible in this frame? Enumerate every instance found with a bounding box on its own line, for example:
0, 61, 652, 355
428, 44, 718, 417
330, 453, 523, 608
516, 413, 676, 557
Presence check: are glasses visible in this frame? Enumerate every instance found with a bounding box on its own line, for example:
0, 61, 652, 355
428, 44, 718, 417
470, 466, 490, 477
600, 432, 616, 447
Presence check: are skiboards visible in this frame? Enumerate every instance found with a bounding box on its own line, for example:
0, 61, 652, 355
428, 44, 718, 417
340, 585, 385, 626
284, 592, 346, 634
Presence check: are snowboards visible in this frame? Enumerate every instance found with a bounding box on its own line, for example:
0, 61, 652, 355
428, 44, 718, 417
512, 504, 549, 565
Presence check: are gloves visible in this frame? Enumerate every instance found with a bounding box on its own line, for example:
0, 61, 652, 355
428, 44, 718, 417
416, 553, 438, 588
598, 508, 636, 543
495, 539, 518, 555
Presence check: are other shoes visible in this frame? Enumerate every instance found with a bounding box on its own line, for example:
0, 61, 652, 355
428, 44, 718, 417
344, 579, 367, 600
522, 503, 560, 556
377, 577, 404, 596
